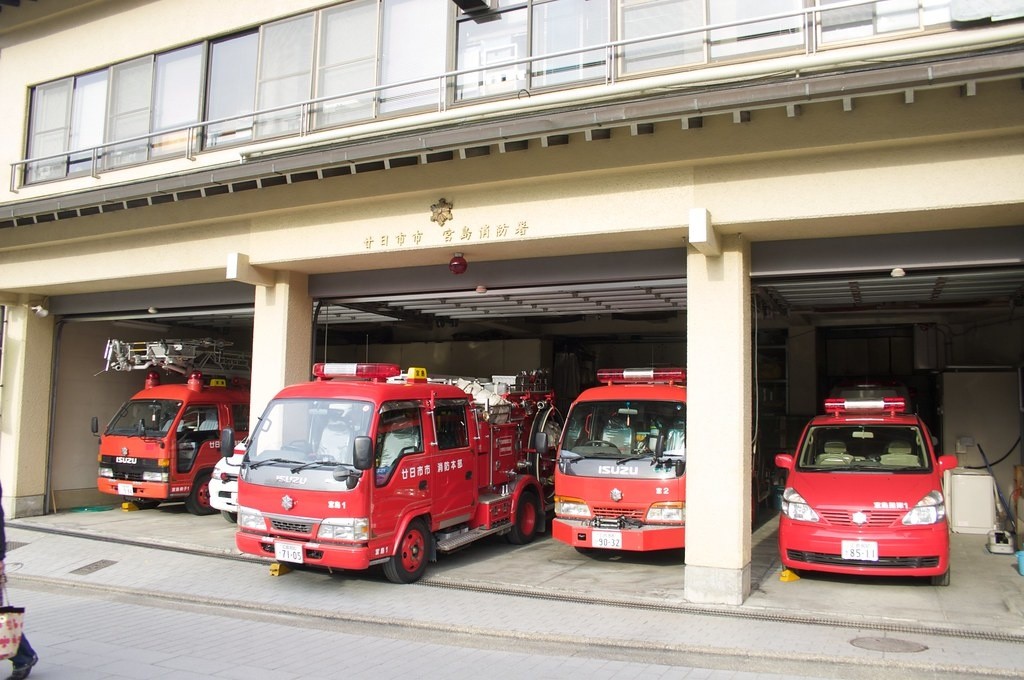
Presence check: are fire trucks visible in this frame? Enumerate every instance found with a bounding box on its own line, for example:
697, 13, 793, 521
236, 361, 564, 585
552, 368, 771, 552
90, 338, 250, 516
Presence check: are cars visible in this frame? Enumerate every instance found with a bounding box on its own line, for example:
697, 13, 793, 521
208, 437, 247, 523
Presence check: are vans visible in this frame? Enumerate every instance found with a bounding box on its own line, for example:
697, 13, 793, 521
774, 396, 958, 587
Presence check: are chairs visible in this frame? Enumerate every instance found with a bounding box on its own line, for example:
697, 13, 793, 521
878, 440, 921, 466
662, 415, 685, 456
602, 415, 633, 456
159, 413, 185, 432
815, 441, 854, 465
314, 413, 354, 465
380, 410, 421, 468
198, 408, 218, 431
